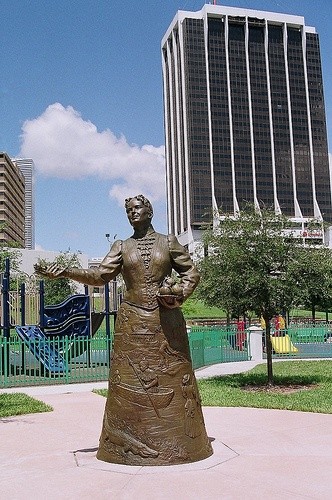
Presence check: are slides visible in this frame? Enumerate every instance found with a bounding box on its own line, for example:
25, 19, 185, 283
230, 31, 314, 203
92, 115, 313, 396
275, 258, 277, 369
269, 334, 298, 354
58, 312, 105, 361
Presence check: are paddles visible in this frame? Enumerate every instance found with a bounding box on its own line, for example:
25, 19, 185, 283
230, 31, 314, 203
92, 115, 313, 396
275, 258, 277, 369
109, 377, 122, 407
126, 355, 160, 418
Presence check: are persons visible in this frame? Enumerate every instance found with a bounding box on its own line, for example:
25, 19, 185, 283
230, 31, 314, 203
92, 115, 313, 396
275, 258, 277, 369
31, 194, 213, 465
